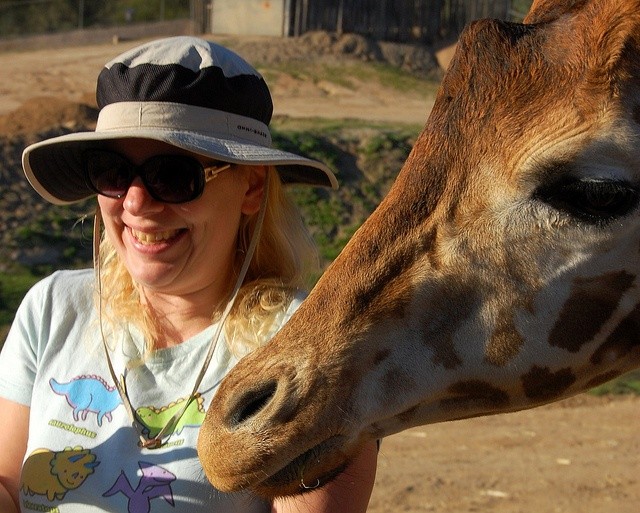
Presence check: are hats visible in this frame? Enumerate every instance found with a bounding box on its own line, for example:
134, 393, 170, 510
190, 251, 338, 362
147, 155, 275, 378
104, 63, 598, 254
22, 33, 341, 209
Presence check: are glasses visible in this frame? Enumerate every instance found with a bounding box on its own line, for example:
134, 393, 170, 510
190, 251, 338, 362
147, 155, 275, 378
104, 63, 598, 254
78, 146, 239, 205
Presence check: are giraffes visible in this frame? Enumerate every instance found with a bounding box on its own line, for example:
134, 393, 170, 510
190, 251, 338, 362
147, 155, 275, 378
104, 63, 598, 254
192, 1, 639, 513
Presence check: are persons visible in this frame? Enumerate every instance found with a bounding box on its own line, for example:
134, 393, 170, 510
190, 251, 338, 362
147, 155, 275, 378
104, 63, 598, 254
1, 35, 379, 513
